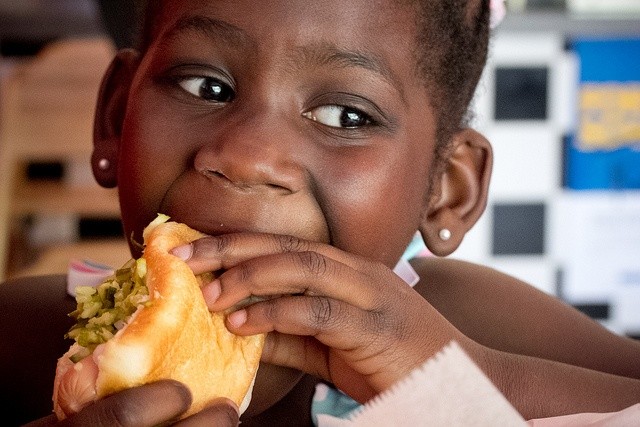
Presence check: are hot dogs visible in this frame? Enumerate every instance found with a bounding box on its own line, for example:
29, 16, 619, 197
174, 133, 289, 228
52, 212, 267, 418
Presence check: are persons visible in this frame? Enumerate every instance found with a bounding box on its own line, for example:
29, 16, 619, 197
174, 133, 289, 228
0, 0, 636, 427
